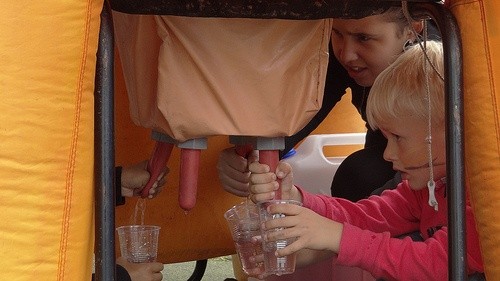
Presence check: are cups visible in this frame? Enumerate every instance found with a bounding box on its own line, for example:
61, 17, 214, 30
116, 224, 162, 263
255, 199, 303, 274
223, 201, 267, 276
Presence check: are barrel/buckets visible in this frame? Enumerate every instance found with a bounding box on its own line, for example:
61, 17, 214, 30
279, 132, 369, 197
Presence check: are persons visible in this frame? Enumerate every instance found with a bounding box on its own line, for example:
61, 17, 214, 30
92, 158, 169, 281
217, 14, 440, 202
248, 41, 486, 281
